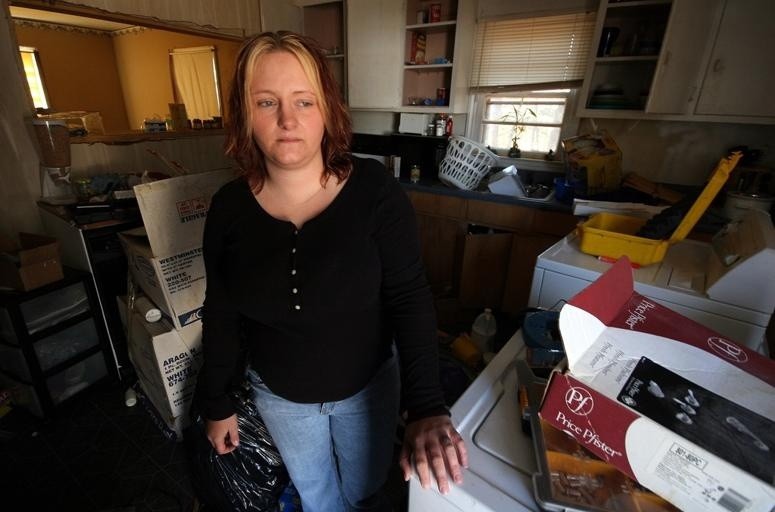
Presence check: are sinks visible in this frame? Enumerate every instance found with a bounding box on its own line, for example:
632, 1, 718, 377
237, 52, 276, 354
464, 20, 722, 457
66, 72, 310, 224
431, 183, 488, 196
489, 185, 556, 205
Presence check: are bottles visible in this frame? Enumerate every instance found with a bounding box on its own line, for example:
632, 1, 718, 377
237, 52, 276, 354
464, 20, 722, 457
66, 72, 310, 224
446, 116, 453, 136
426, 124, 436, 136
469, 307, 497, 346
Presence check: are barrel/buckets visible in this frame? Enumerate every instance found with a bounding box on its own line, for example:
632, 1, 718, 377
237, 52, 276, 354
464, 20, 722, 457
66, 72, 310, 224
471, 308, 496, 354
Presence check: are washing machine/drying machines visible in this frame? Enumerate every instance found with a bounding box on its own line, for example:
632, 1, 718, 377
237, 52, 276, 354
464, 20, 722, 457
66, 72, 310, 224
525, 208, 775, 358
405, 329, 545, 512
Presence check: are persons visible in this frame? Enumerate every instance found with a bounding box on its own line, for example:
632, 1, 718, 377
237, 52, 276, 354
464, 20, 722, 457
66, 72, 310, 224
193, 30, 471, 511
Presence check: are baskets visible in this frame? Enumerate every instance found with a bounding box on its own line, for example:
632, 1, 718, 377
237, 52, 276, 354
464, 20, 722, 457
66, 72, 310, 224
438, 135, 500, 192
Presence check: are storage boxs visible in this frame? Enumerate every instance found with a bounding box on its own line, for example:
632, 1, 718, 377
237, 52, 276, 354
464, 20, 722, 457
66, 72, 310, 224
0, 233, 64, 292
116, 295, 204, 442
540, 256, 775, 512
118, 168, 238, 326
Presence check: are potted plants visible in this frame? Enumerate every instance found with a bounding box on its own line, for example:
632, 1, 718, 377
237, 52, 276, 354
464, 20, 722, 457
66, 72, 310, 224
499, 97, 538, 157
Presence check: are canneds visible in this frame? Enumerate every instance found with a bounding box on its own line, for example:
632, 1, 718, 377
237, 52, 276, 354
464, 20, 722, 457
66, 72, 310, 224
410, 165, 420, 184
437, 88, 446, 106
416, 7, 427, 23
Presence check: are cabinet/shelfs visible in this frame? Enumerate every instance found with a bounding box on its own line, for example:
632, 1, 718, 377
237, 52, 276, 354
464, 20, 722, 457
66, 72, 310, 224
406, 192, 581, 319
300, 0, 476, 113
576, 0, 775, 125
0, 273, 119, 424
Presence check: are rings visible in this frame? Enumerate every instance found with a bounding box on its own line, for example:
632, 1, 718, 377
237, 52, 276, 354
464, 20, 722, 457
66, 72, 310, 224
440, 436, 453, 451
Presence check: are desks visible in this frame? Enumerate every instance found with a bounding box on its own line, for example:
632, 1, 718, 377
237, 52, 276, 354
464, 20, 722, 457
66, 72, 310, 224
39, 201, 141, 383
